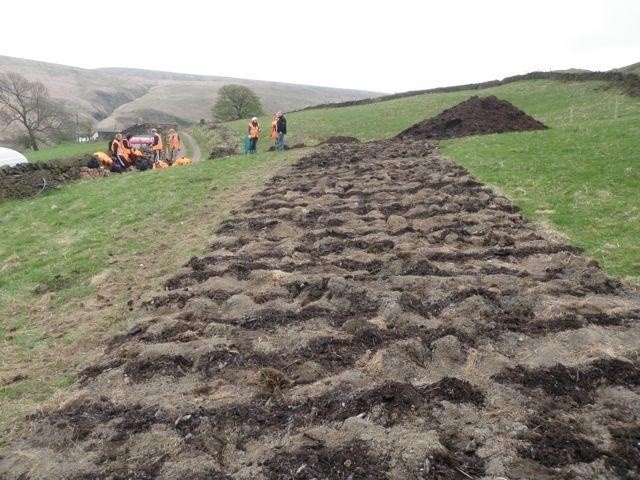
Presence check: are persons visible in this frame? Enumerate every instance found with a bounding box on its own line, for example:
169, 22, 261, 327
248, 117, 259, 153
111, 128, 180, 170
272, 111, 286, 151
